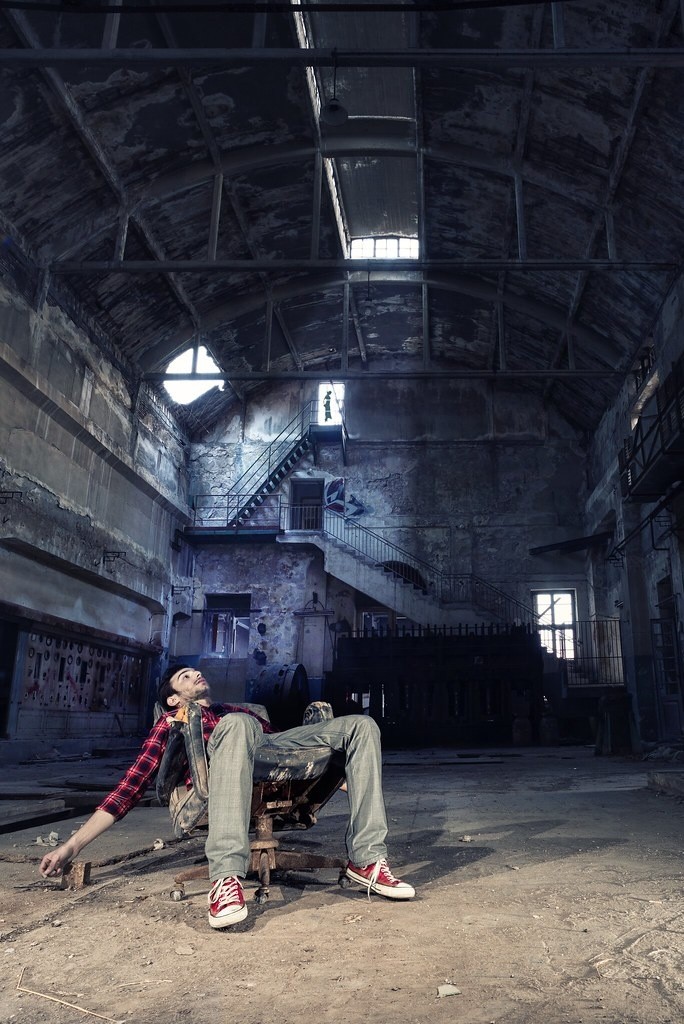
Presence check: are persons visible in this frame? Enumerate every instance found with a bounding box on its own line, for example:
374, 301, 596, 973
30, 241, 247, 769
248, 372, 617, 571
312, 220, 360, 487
39, 665, 415, 930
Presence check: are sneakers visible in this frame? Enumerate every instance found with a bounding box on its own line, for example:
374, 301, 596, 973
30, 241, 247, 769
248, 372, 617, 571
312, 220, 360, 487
208, 876, 248, 927
346, 860, 415, 900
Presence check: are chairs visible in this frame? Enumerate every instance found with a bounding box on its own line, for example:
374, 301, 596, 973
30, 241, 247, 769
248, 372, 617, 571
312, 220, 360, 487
153, 701, 348, 905
